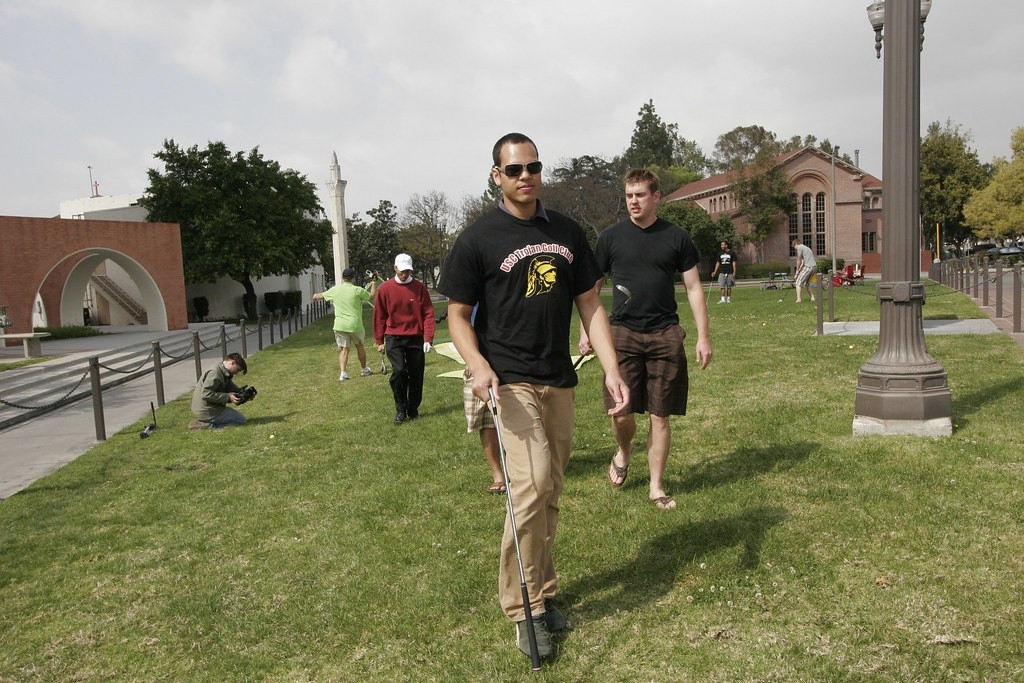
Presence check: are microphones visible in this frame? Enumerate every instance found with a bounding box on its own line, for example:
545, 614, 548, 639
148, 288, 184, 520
237, 385, 248, 393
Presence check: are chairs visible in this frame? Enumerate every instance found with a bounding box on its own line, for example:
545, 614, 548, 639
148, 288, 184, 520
833, 263, 865, 287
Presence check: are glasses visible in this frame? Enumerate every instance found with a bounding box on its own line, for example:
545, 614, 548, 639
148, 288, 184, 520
496, 160, 544, 177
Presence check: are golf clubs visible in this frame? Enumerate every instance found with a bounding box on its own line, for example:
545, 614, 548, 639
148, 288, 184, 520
380, 354, 387, 374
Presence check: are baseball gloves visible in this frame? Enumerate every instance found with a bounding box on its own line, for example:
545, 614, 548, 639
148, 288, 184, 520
236, 385, 257, 405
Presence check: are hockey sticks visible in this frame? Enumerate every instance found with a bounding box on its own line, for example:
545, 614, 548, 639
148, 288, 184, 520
704, 276, 715, 305
572, 285, 634, 375
482, 384, 543, 673
780, 274, 798, 301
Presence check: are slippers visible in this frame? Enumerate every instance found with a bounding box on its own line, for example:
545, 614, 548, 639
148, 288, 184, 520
608, 452, 630, 487
489, 481, 506, 493
652, 495, 678, 512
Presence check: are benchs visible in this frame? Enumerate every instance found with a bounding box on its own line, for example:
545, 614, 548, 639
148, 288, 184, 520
0, 332, 52, 358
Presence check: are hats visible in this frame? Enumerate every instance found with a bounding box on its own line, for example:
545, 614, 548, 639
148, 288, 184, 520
395, 253, 414, 272
342, 268, 356, 279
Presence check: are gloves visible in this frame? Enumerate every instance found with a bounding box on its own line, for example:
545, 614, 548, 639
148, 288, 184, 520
423, 342, 433, 353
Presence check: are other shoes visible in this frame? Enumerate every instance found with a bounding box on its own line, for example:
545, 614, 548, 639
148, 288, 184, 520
726, 299, 731, 304
716, 299, 727, 305
394, 411, 407, 424
407, 409, 419, 419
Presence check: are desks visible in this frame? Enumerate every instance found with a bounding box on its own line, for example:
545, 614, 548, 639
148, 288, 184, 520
760, 281, 796, 290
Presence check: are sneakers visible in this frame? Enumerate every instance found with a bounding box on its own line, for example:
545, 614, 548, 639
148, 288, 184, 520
541, 599, 566, 633
516, 614, 552, 657
360, 367, 372, 376
339, 373, 351, 381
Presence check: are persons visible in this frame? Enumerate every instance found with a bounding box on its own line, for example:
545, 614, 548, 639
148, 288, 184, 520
462, 302, 506, 494
792, 239, 818, 303
711, 241, 737, 304
579, 169, 713, 511
313, 269, 376, 382
434, 133, 630, 659
373, 253, 436, 426
187, 353, 247, 430
